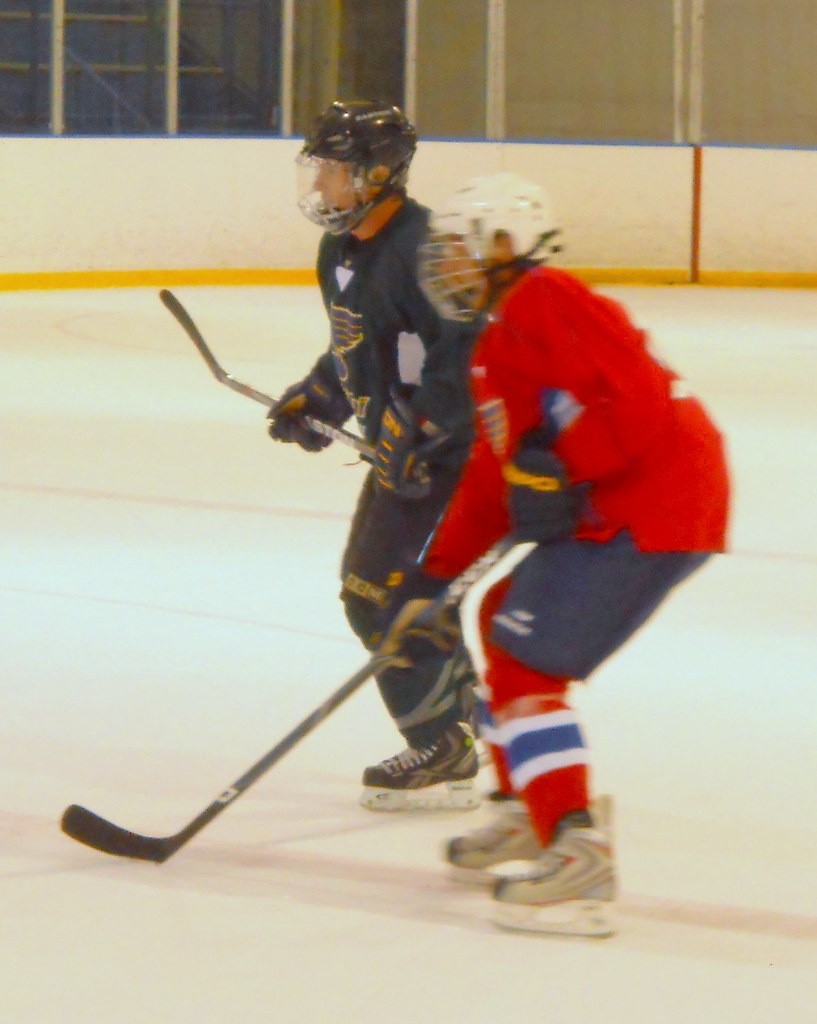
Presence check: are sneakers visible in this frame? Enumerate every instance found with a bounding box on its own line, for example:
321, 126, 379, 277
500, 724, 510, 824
487, 792, 620, 936
358, 684, 483, 809
448, 792, 539, 890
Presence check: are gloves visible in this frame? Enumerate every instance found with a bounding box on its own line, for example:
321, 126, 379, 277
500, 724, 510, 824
266, 354, 354, 453
377, 383, 468, 500
371, 564, 462, 673
504, 427, 574, 540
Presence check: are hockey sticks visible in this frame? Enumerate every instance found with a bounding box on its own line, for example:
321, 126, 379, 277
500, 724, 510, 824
59, 530, 523, 866
158, 289, 439, 484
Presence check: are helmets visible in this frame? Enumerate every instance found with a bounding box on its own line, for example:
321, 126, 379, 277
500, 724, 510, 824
415, 169, 561, 321
295, 98, 418, 233
